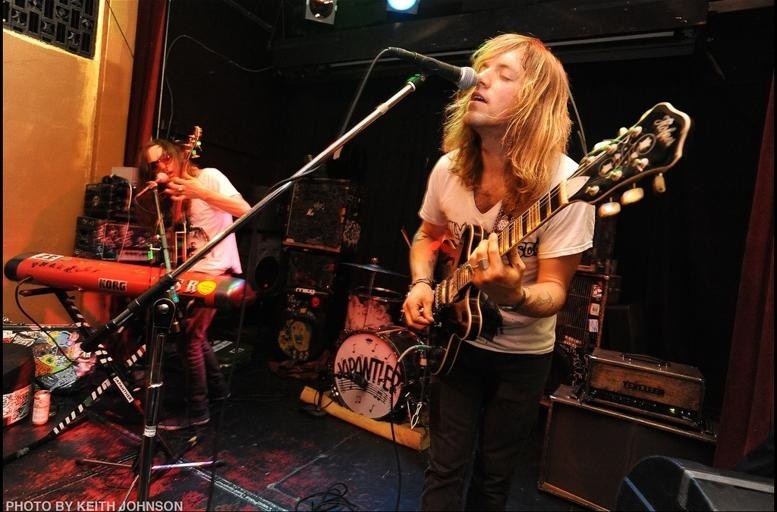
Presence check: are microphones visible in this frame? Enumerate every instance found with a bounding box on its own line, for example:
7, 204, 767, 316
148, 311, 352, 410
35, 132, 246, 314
134, 171, 170, 199
387, 46, 479, 90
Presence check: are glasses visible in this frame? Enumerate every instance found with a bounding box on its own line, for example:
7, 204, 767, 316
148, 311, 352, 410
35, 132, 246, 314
147, 152, 171, 167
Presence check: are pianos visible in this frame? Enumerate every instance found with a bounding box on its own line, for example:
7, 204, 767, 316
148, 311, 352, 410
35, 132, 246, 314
4, 251, 257, 307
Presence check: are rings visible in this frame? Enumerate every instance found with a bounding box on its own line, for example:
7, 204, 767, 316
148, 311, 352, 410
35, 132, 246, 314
467, 264, 477, 276
178, 187, 182, 191
477, 259, 488, 269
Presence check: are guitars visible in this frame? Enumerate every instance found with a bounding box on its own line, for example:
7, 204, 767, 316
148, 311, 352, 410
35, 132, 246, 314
165, 125, 203, 270
420, 101, 692, 379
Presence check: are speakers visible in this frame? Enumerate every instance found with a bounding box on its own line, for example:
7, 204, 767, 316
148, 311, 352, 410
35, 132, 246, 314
281, 176, 361, 254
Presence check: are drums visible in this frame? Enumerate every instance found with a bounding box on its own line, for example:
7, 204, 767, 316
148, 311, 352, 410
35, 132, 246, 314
343, 285, 404, 329
331, 325, 430, 429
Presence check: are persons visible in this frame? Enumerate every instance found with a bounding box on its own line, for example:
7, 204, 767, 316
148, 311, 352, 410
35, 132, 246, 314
138, 138, 252, 431
404, 33, 595, 511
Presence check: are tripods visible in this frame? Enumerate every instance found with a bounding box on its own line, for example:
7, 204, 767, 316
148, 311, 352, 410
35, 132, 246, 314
76, 192, 228, 506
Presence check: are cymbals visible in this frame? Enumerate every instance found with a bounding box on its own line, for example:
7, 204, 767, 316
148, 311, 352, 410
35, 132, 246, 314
341, 256, 410, 278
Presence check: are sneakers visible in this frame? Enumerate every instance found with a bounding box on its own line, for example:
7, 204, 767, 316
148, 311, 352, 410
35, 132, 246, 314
184, 390, 231, 401
157, 411, 214, 430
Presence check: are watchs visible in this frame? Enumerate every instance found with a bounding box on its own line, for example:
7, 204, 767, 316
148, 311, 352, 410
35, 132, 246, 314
497, 288, 527, 312
